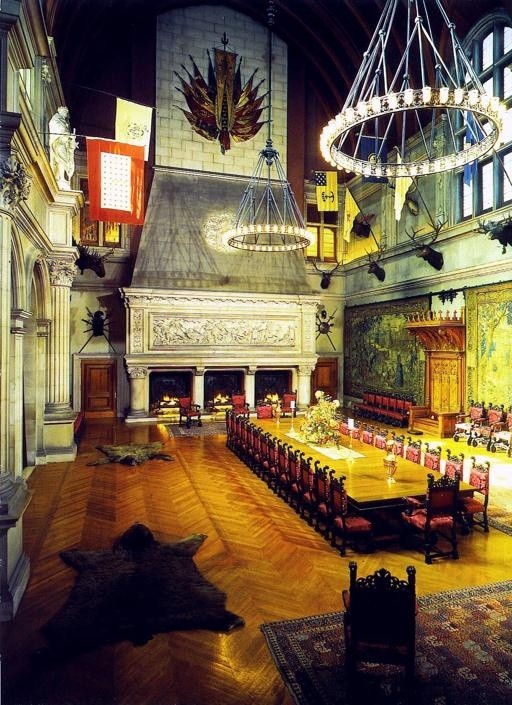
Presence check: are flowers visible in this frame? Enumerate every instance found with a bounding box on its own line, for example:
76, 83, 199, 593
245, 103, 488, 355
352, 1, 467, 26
299, 390, 342, 449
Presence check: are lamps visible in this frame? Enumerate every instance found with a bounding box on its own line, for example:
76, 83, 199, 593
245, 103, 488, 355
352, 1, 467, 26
221, 0, 317, 253
318, 0, 507, 179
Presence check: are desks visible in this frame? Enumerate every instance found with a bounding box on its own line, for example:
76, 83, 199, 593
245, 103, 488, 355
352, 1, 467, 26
244, 416, 481, 537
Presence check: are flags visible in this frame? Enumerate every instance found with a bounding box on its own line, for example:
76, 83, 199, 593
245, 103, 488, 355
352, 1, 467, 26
315, 170, 339, 212
393, 152, 413, 221
85, 135, 145, 225
463, 111, 488, 187
114, 96, 153, 162
342, 187, 360, 243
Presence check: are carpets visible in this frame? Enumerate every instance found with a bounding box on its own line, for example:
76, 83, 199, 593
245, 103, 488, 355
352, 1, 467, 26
258, 578, 511, 705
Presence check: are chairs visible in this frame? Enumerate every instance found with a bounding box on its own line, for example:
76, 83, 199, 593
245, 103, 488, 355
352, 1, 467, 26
179, 396, 202, 429
339, 414, 491, 533
224, 407, 372, 560
230, 394, 251, 420
468, 402, 505, 451
256, 399, 273, 419
281, 392, 298, 418
451, 399, 485, 445
342, 559, 418, 679
350, 391, 413, 430
399, 470, 463, 564
490, 406, 511, 458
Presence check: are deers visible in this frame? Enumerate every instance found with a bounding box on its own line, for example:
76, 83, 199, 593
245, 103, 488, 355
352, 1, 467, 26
309, 257, 345, 289
471, 214, 512, 248
404, 218, 450, 270
363, 244, 389, 281
73, 242, 118, 278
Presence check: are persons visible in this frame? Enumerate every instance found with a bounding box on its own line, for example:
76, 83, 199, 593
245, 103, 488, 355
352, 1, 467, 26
49, 105, 77, 192
